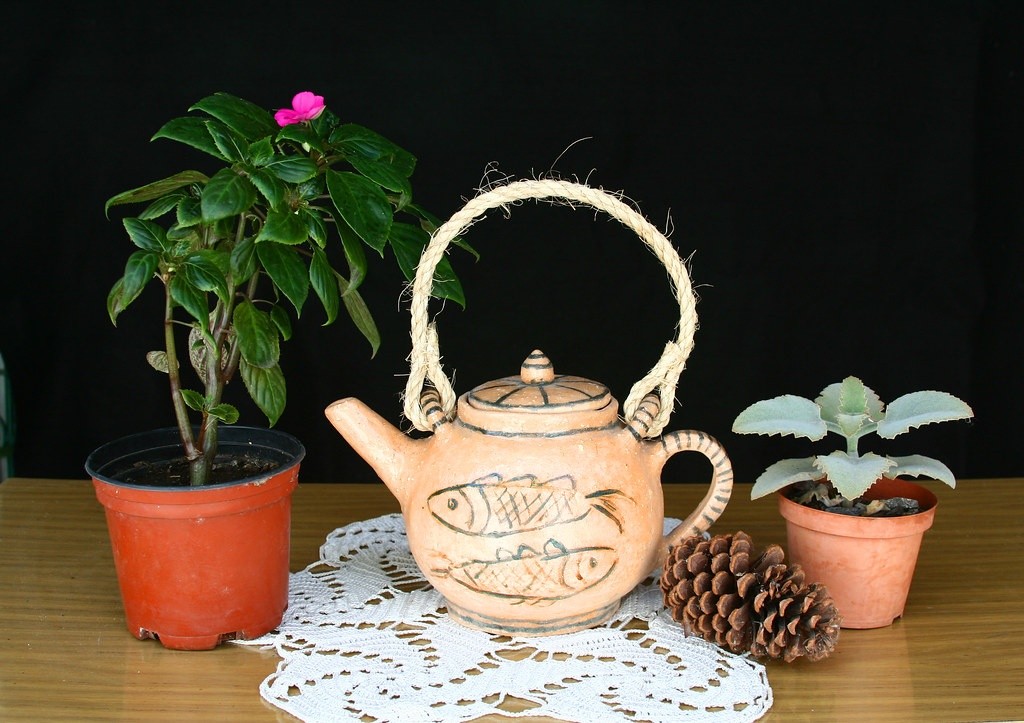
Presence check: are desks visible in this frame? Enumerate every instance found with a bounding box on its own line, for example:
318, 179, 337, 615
0, 477, 1024, 723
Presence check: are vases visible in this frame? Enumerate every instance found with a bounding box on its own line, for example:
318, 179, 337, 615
82, 423, 307, 652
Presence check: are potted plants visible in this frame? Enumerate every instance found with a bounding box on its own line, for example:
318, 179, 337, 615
726, 372, 974, 629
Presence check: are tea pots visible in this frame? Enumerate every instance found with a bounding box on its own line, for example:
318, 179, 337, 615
324, 179, 733, 634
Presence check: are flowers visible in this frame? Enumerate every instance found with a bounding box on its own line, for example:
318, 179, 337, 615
111, 86, 485, 486
